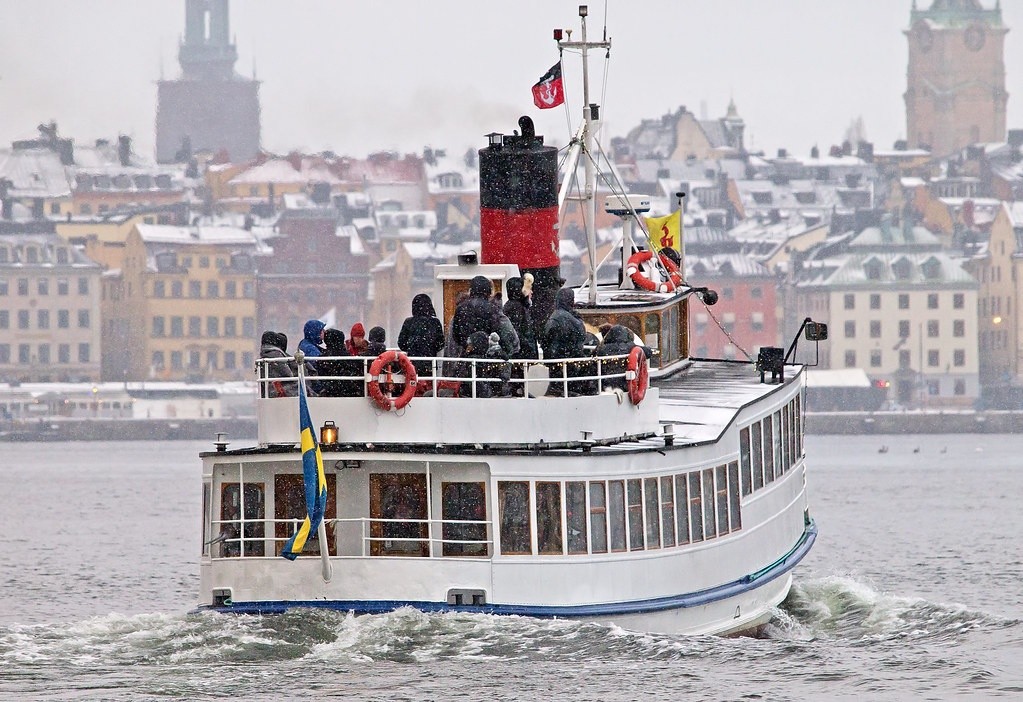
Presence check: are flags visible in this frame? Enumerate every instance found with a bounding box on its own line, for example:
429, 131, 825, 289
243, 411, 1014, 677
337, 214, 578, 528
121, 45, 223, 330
645, 210, 681, 256
532, 59, 564, 109
280, 376, 328, 561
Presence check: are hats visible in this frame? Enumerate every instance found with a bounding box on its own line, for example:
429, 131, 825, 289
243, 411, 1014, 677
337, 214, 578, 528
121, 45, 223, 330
351, 323, 365, 337
488, 332, 500, 347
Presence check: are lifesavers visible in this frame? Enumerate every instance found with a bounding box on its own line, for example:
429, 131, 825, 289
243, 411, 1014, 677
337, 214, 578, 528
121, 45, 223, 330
368, 350, 419, 413
626, 346, 650, 405
626, 249, 681, 293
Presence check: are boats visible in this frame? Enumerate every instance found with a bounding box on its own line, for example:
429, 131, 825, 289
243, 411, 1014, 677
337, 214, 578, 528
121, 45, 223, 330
195, 6, 829, 640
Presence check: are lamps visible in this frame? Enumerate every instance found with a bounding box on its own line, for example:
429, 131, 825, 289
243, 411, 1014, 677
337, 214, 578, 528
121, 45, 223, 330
693, 286, 719, 305
320, 419, 339, 444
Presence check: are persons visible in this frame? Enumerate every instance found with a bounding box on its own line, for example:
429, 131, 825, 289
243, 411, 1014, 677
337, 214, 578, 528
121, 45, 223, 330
297, 320, 326, 370
260, 331, 325, 398
398, 293, 444, 377
542, 289, 652, 398
446, 273, 539, 400
315, 323, 401, 397
